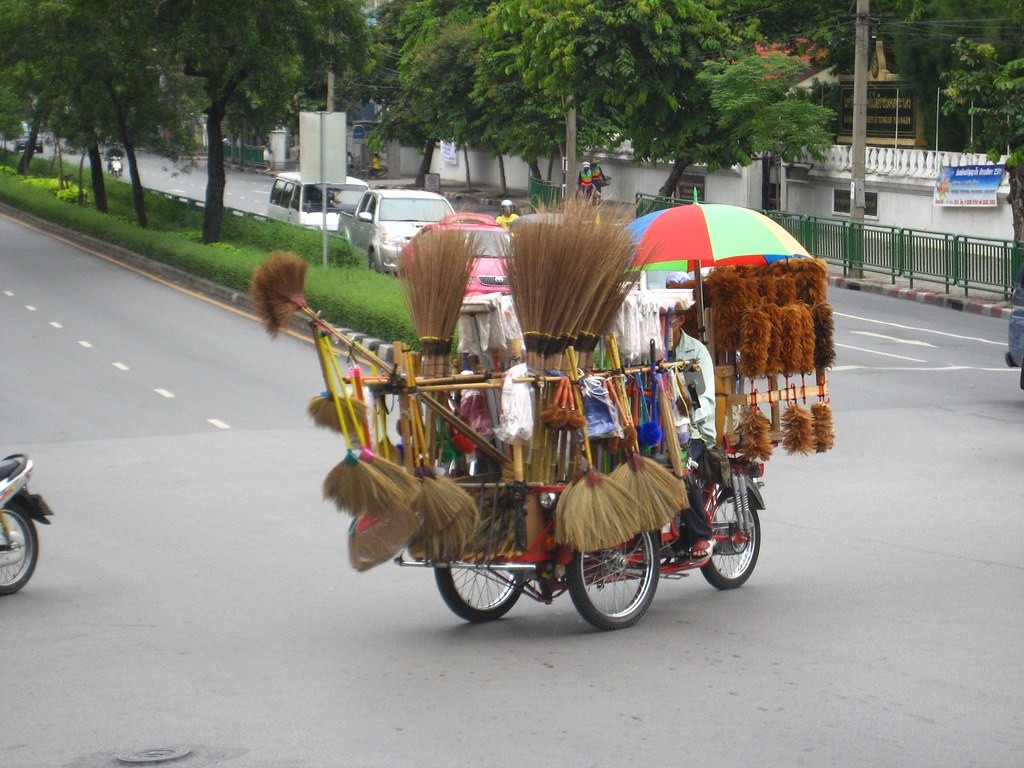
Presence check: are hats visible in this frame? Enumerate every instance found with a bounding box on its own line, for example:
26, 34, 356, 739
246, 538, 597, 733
590, 158, 600, 165
583, 161, 590, 168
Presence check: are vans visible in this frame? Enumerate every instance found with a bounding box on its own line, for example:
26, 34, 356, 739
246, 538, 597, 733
267, 172, 372, 232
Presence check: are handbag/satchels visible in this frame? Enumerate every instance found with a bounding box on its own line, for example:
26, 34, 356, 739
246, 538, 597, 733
457, 388, 494, 442
494, 362, 533, 444
591, 184, 601, 199
575, 367, 625, 442
600, 174, 611, 186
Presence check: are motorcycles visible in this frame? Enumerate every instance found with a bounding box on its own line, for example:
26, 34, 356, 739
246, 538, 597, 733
0, 454, 54, 595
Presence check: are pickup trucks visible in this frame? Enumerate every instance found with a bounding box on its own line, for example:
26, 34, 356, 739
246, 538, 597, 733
336, 188, 458, 277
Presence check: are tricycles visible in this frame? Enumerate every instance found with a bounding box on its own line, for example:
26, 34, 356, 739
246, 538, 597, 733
395, 457, 766, 631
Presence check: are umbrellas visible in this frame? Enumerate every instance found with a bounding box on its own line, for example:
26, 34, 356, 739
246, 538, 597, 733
621, 187, 814, 347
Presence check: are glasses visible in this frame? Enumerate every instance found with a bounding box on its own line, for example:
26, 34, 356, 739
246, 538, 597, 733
659, 311, 678, 320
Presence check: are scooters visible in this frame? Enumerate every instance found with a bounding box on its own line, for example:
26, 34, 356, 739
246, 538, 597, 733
105, 155, 122, 178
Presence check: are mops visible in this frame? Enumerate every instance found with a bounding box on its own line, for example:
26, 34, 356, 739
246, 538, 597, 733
456, 292, 528, 475
595, 289, 680, 544
492, 362, 534, 499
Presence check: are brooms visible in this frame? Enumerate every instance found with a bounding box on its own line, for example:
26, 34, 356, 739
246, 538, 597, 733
383, 196, 500, 469
459, 332, 689, 566
489, 181, 668, 485
306, 321, 480, 573
249, 251, 513, 469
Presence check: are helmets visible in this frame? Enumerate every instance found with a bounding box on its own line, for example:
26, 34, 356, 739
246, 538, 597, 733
501, 200, 513, 214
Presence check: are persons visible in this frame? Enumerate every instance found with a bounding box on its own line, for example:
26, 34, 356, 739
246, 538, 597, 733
368, 152, 380, 179
496, 199, 520, 237
346, 151, 354, 176
578, 159, 606, 205
222, 135, 272, 172
105, 143, 124, 176
659, 310, 717, 563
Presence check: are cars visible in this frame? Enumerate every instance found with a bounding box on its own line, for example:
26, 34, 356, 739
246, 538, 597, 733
395, 213, 519, 297
14, 121, 43, 153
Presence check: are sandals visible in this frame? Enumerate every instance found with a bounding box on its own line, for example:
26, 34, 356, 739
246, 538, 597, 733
689, 537, 717, 562
